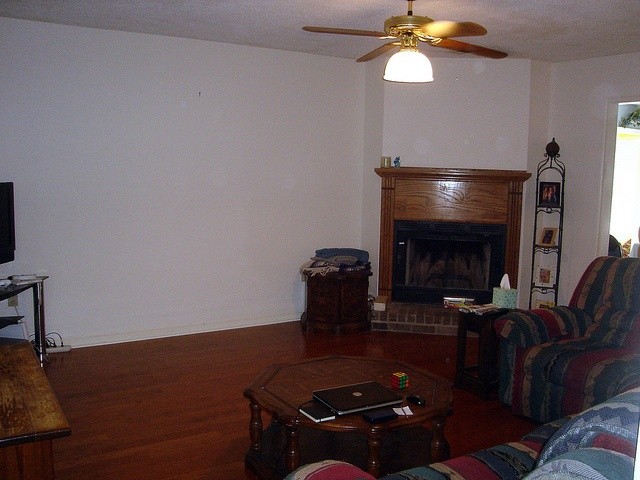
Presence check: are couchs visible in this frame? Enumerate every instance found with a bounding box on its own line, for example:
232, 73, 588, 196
285, 385, 640, 480
494, 255, 639, 422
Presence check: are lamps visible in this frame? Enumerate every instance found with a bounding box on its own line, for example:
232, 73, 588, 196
382, 33, 435, 83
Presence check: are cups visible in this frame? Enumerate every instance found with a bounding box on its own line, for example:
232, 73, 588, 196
381, 155, 393, 168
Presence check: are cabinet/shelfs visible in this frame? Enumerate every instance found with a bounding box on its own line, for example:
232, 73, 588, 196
301, 272, 373, 332
529, 137, 565, 310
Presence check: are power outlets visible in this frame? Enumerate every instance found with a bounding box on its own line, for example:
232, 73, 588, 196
8, 294, 18, 307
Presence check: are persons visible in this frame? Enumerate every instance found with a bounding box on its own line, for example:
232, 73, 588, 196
540, 270, 548, 283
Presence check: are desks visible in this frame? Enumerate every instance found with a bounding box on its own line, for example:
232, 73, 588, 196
0, 276, 49, 362
1, 337, 72, 480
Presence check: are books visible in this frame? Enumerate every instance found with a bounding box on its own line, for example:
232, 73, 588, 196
299, 407, 335, 424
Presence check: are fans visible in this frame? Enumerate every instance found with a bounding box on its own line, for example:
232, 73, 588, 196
301, 0, 508, 62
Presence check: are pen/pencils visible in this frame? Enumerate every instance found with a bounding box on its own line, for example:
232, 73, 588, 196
399, 404, 408, 419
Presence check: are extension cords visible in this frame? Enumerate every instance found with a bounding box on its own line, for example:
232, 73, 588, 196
44, 345, 72, 354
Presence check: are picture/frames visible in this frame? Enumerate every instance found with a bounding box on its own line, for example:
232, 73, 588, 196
535, 264, 554, 288
535, 299, 555, 309
539, 182, 560, 206
539, 227, 557, 246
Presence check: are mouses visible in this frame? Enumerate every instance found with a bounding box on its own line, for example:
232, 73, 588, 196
407, 394, 427, 408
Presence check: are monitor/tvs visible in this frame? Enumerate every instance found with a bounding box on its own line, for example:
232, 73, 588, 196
0, 180, 16, 266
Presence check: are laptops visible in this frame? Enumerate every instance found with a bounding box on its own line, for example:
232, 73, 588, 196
311, 381, 405, 417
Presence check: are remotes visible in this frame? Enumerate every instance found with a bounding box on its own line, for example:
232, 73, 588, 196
483, 308, 511, 320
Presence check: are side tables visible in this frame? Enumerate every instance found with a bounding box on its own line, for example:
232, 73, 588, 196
455, 308, 510, 391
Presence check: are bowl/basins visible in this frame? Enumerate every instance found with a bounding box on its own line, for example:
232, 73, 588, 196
443, 297, 473, 309
